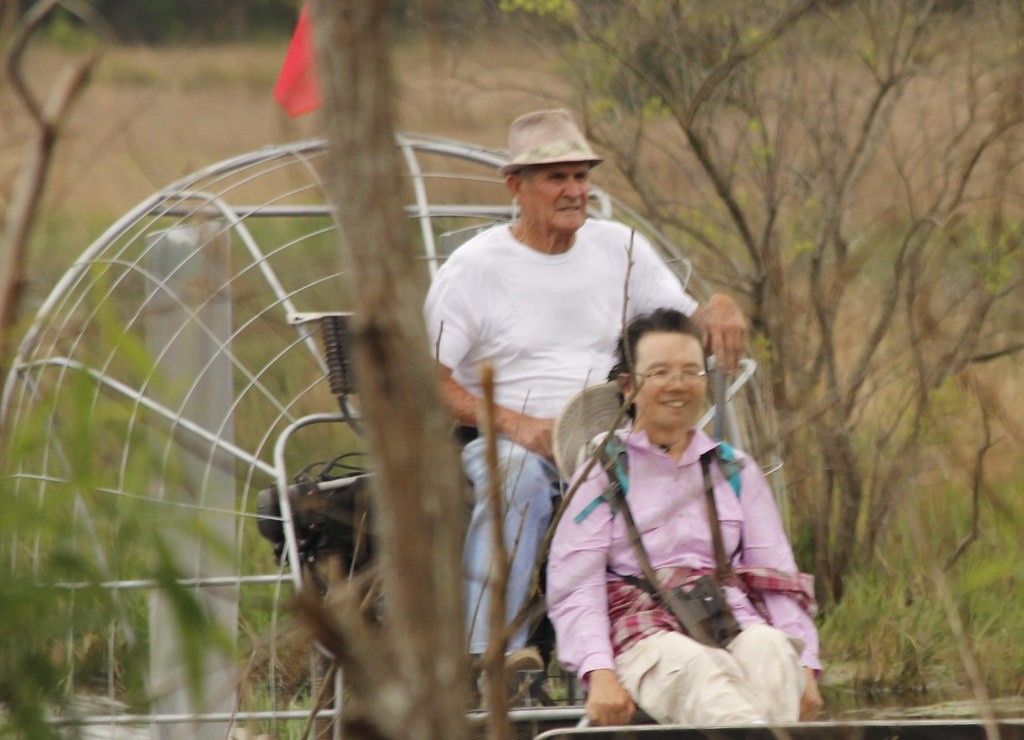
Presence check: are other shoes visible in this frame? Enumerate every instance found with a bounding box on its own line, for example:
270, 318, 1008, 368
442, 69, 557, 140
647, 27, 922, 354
477, 649, 545, 697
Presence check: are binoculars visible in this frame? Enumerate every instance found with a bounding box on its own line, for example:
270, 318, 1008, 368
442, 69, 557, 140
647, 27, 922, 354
668, 572, 743, 642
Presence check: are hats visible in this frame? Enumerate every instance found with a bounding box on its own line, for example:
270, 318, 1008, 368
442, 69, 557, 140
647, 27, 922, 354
552, 381, 717, 486
500, 109, 605, 176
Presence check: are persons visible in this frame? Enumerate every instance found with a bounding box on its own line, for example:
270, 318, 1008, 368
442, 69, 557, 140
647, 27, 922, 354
426, 106, 749, 690
548, 308, 826, 727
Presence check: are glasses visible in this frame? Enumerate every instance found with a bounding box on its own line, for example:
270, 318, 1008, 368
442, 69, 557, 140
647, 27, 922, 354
634, 369, 710, 386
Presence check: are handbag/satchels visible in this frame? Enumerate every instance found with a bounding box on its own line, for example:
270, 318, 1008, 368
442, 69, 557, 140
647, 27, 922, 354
664, 575, 742, 647
